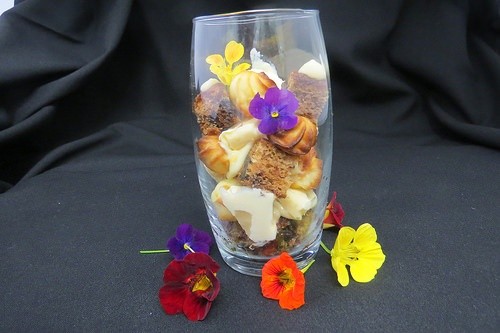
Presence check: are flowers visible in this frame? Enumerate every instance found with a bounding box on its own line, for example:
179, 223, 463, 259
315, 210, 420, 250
139, 223, 212, 259
260, 252, 316, 311
320, 222, 386, 287
159, 253, 222, 321
323, 192, 345, 229
205, 40, 251, 88
248, 87, 300, 135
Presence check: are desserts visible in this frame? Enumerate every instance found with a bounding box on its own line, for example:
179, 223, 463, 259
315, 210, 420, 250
191, 59, 329, 255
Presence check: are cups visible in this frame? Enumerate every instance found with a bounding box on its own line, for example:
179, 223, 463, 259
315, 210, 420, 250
190, 9, 334, 277
